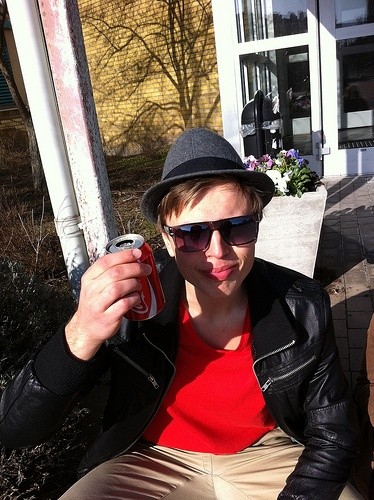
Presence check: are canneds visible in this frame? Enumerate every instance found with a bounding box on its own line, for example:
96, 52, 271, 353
104, 234, 167, 320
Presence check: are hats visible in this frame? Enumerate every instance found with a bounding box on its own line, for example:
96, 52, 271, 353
141, 128, 276, 223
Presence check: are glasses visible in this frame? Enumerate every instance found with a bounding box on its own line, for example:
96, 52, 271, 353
164, 212, 261, 252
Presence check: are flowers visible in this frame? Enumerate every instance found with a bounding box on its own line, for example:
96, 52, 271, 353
244, 149, 322, 198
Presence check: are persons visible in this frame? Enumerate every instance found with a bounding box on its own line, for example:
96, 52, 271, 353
354, 306, 374, 500
0, 127, 362, 500
